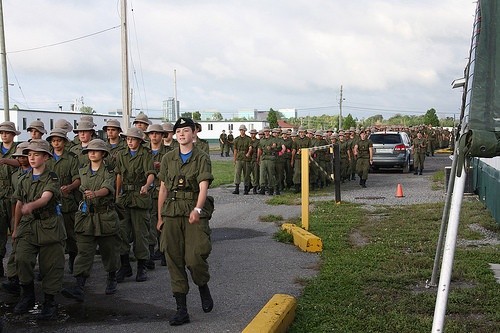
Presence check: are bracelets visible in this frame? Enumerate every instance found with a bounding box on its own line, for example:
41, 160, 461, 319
94, 193, 97, 197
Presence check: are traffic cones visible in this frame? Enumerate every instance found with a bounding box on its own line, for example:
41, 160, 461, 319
395, 183, 405, 197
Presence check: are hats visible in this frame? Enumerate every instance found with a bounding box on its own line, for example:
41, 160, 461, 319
222, 130, 233, 133
143, 118, 202, 133
46, 128, 69, 144
103, 119, 122, 132
22, 139, 53, 160
76, 116, 97, 126
0, 121, 21, 135
73, 121, 95, 134
11, 142, 30, 159
54, 119, 73, 132
239, 123, 457, 141
82, 139, 110, 158
133, 114, 152, 125
27, 120, 47, 134
120, 128, 147, 144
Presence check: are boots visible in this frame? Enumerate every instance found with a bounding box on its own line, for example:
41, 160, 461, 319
232, 172, 356, 196
414, 167, 418, 174
0, 244, 166, 316
419, 167, 424, 175
359, 176, 367, 188
199, 285, 213, 313
170, 293, 190, 325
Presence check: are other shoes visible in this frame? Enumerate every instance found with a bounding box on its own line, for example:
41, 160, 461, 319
221, 152, 234, 157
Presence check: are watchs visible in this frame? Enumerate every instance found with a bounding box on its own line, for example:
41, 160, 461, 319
194, 208, 202, 215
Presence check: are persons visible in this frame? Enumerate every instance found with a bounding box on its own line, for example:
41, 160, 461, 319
0, 114, 210, 317
375, 124, 458, 176
219, 125, 374, 195
155, 117, 215, 326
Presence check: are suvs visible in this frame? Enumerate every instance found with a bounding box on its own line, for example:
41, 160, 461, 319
368, 129, 413, 173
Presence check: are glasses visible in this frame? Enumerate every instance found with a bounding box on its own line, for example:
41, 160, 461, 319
125, 137, 137, 141
